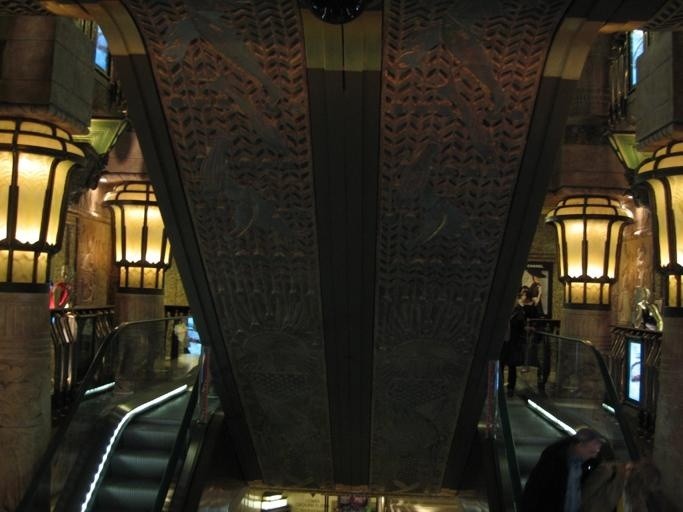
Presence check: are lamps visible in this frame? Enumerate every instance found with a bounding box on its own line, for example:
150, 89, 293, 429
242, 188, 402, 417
0, 115, 85, 294
634, 139, 683, 318
544, 196, 636, 310
100, 180, 174, 295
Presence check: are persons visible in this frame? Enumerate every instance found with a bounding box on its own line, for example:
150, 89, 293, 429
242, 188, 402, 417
499, 282, 663, 398
579, 452, 662, 512
519, 426, 615, 512
